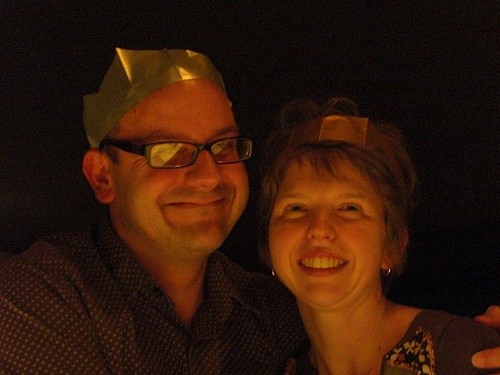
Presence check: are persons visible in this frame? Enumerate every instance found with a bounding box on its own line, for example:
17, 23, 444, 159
2, 46, 499, 374
256, 97, 499, 374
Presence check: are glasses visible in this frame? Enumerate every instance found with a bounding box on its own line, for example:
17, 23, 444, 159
102, 133, 253, 169
